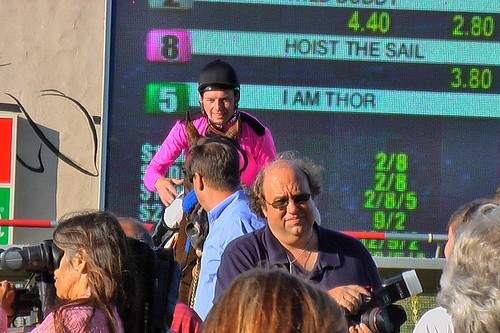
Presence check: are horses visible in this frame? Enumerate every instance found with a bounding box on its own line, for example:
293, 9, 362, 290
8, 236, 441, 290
149, 111, 241, 301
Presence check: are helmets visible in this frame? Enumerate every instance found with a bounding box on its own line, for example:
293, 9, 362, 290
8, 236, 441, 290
198, 59, 240, 91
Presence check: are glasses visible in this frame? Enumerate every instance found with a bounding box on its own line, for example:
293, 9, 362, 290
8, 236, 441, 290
188, 173, 197, 183
264, 192, 313, 210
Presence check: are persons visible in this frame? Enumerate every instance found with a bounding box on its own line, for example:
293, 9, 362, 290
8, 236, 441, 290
186, 137, 266, 321
144, 61, 276, 303
197, 267, 348, 333
116, 217, 201, 333
212, 159, 383, 333
410, 198, 500, 333
0, 211, 134, 333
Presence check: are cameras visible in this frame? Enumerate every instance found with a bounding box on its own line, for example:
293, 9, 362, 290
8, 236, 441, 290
347, 270, 423, 333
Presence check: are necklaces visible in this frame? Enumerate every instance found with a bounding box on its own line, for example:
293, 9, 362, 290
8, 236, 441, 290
287, 234, 312, 274
